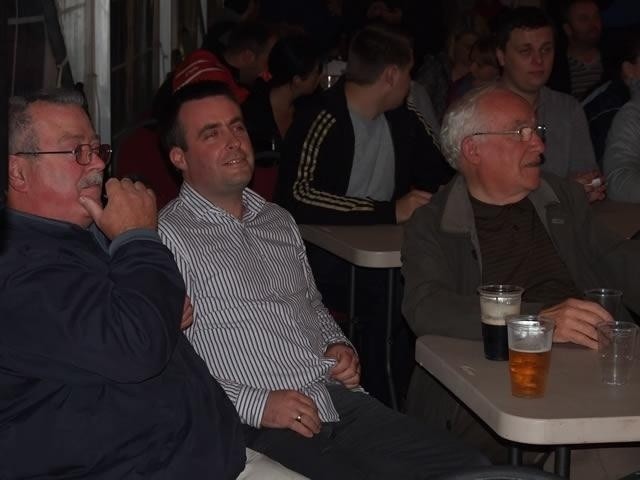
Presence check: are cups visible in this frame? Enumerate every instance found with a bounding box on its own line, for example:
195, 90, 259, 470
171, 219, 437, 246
584, 288, 622, 326
478, 285, 525, 362
505, 315, 557, 396
595, 320, 636, 385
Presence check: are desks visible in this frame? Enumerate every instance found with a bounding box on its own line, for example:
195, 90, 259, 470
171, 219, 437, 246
413, 332, 640, 479
295, 220, 408, 371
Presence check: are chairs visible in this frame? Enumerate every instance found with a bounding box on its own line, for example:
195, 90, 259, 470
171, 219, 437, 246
247, 165, 277, 202
107, 115, 180, 212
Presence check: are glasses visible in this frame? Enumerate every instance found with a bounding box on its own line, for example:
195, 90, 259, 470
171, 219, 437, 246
14, 142, 111, 167
469, 121, 552, 142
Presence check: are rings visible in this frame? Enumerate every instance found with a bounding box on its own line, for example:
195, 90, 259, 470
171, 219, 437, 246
297, 414, 304, 421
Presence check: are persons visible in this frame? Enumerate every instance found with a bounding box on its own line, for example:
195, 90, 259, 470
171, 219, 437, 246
143, 79, 496, 479
397, 81, 640, 479
1, 86, 320, 477
